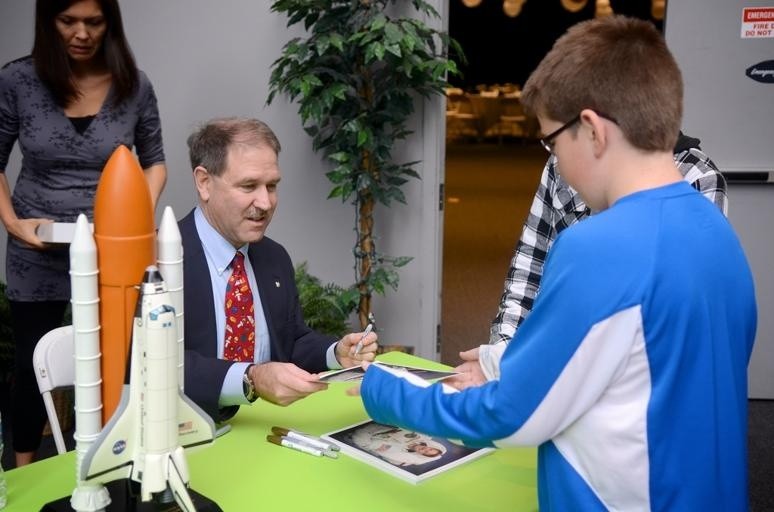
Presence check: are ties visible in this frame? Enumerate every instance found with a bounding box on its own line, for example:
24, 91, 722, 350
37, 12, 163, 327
223, 251, 256, 363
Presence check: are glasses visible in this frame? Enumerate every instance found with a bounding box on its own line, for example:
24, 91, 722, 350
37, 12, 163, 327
539, 109, 620, 156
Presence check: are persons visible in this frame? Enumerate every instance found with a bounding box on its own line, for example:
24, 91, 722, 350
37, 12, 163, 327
489, 129, 729, 347
156, 115, 378, 424
348, 423, 447, 466
343, 15, 757, 512
0, 0, 168, 467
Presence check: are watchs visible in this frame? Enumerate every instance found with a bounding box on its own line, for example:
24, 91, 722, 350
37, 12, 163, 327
242, 364, 256, 403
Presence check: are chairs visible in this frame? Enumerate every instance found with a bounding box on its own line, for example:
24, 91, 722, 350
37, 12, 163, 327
32, 325, 78, 454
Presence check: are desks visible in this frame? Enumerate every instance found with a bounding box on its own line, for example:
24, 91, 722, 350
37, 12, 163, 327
0, 349, 540, 512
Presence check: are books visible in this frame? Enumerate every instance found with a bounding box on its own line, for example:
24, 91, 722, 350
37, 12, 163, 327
319, 419, 496, 486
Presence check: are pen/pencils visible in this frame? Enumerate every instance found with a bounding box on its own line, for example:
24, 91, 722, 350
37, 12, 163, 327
354, 318, 376, 357
266, 427, 340, 459
